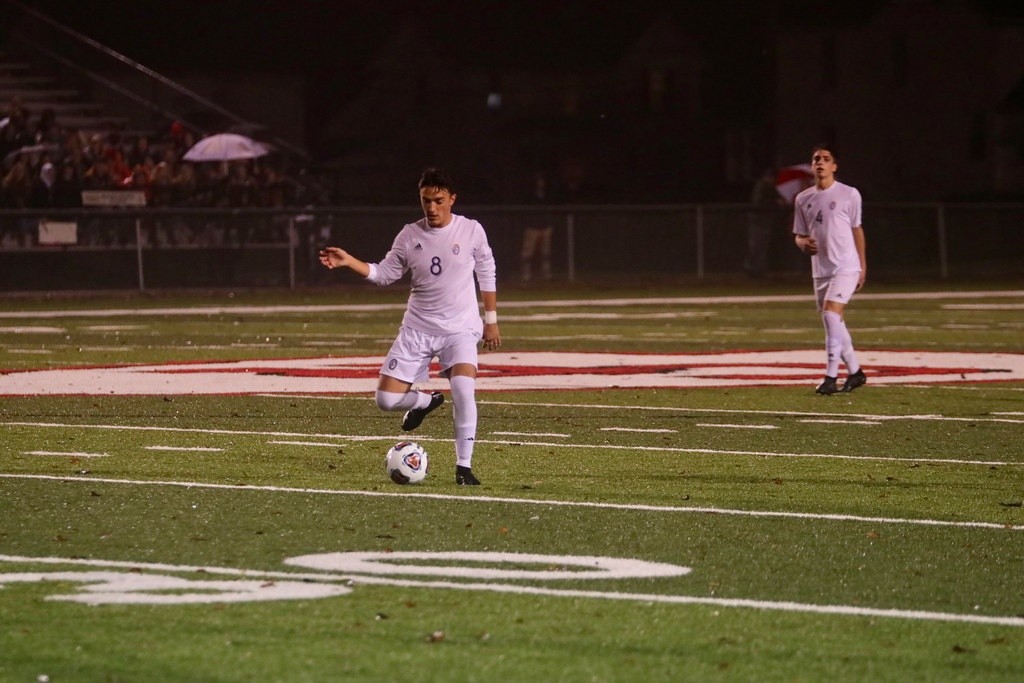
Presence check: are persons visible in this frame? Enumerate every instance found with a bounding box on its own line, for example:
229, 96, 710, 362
319, 168, 502, 486
792, 146, 867, 395
0, 92, 334, 247
521, 170, 556, 291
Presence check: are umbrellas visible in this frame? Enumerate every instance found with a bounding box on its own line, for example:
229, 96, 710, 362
775, 164, 817, 204
183, 130, 270, 177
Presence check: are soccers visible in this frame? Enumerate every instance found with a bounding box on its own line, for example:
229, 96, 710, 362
384, 441, 430, 485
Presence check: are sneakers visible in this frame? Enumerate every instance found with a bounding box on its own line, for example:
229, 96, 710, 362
837, 368, 866, 392
401, 392, 444, 431
455, 472, 481, 485
815, 376, 839, 395
